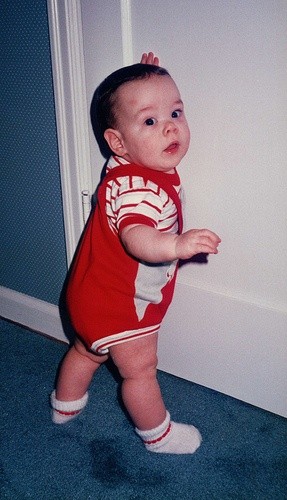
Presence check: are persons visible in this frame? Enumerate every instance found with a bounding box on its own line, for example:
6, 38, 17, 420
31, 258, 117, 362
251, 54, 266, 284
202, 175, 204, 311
48, 50, 223, 456
